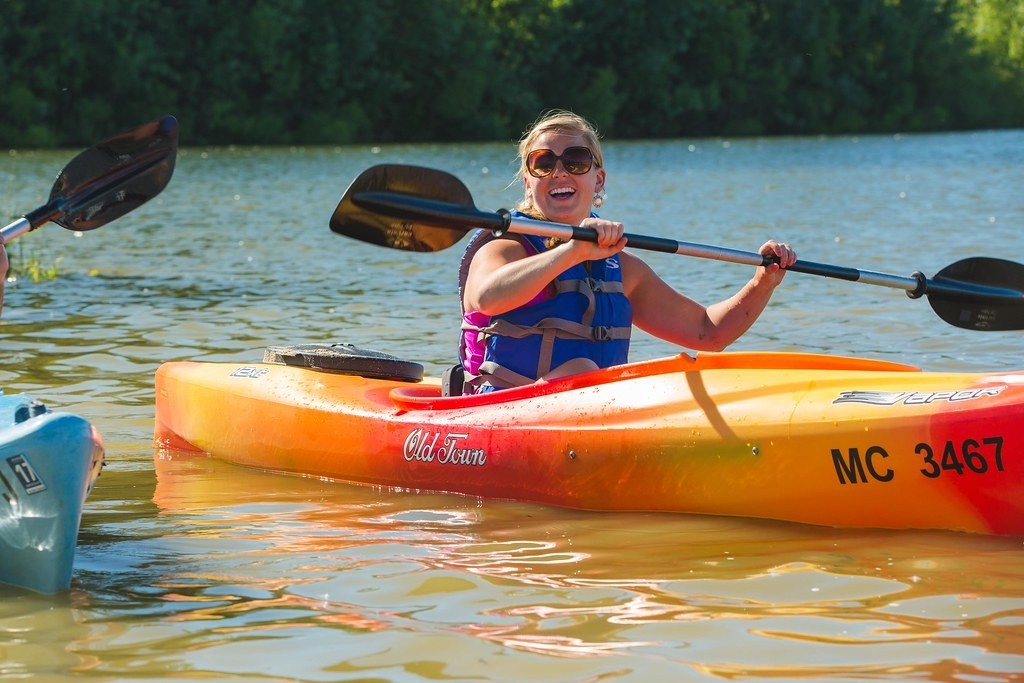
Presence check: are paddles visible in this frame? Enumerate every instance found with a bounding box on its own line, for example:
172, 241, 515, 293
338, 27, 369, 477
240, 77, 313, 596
0, 114, 179, 244
329, 163, 1024, 331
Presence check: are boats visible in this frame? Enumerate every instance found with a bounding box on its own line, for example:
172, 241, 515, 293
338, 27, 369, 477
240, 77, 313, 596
154, 349, 1024, 538
0, 385, 108, 596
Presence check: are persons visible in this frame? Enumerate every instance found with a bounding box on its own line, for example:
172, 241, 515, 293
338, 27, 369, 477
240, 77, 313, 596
457, 108, 796, 397
0, 231, 10, 319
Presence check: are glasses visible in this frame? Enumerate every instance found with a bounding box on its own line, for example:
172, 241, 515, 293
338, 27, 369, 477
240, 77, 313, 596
526, 146, 599, 178
